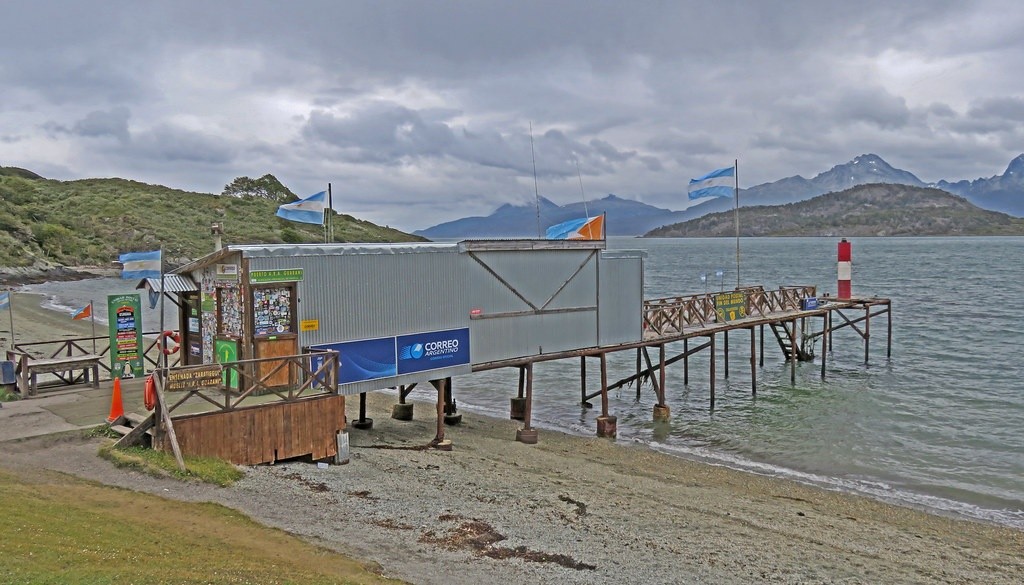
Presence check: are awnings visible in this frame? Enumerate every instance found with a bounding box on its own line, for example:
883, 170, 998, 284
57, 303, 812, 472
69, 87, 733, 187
136, 274, 200, 318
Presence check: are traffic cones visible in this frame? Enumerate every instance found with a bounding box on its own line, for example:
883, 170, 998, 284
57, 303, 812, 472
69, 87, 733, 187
102, 377, 127, 423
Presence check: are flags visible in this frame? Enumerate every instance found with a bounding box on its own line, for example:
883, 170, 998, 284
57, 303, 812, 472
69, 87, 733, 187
687, 166, 735, 202
72, 304, 91, 320
545, 215, 603, 240
274, 190, 327, 225
118, 250, 161, 281
0, 291, 10, 310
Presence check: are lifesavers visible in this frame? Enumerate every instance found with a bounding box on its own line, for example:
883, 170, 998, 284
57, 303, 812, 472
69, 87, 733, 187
157, 330, 181, 356
143, 375, 155, 411
